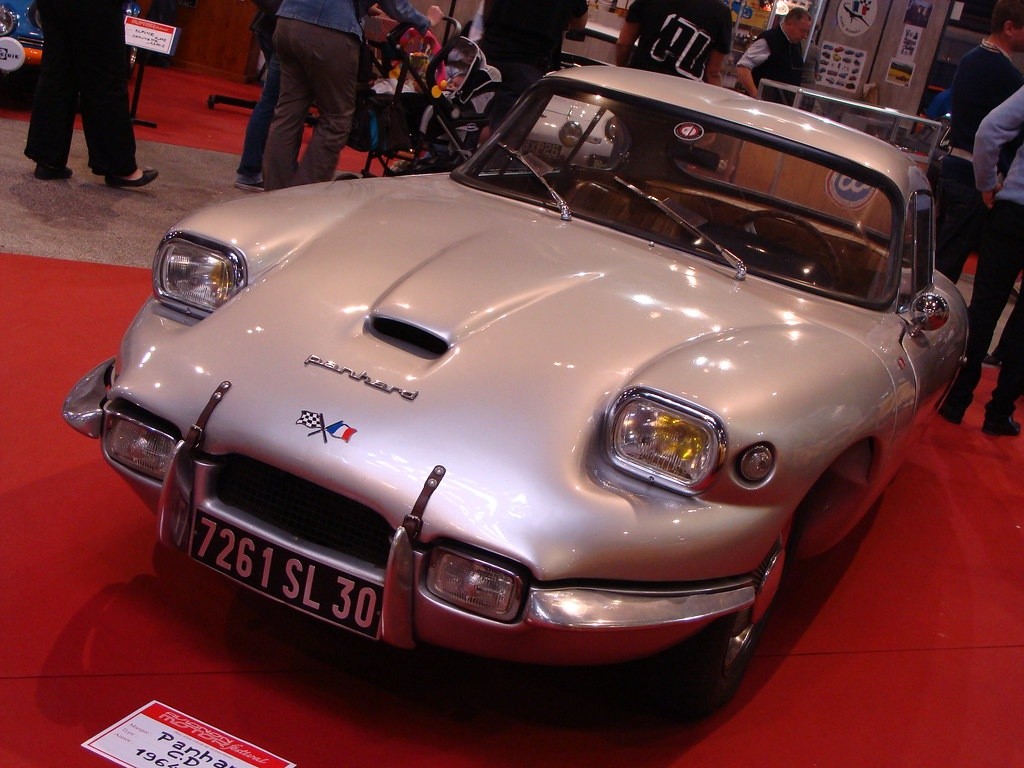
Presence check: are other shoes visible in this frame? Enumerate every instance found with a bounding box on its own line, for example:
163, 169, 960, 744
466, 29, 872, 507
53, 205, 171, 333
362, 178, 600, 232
939, 401, 962, 423
983, 354, 1000, 364
34, 164, 73, 179
105, 169, 159, 186
235, 172, 264, 192
982, 418, 1020, 435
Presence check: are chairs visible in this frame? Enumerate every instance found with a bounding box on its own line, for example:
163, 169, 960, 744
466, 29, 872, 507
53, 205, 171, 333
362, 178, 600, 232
567, 182, 633, 223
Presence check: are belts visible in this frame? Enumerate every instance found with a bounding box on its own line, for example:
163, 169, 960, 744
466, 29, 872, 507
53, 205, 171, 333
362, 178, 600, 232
949, 146, 974, 163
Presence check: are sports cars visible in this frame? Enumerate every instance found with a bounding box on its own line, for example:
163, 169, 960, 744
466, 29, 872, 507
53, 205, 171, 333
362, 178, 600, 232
57, 64, 974, 728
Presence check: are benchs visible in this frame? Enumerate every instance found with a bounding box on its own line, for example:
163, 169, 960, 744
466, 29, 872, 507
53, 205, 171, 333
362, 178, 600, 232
640, 179, 890, 299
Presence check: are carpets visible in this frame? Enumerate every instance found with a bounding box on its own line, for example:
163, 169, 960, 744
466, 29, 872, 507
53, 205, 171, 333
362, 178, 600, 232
1, 249, 1024, 768
0, 60, 425, 177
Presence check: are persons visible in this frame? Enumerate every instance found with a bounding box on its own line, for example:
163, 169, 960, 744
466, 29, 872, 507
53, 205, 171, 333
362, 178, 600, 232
735, 8, 812, 107
615, 0, 732, 87
234, 1, 446, 191
468, 0, 588, 151
25, 0, 159, 188
924, 0, 1024, 436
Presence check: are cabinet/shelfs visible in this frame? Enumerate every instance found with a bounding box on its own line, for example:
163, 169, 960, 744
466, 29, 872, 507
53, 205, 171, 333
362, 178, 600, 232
758, 79, 942, 179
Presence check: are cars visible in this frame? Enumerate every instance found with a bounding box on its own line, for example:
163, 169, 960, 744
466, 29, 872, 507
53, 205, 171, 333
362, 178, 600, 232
490, 51, 631, 176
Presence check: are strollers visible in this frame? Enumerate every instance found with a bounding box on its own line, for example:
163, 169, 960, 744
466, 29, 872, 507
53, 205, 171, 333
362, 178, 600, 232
327, 14, 503, 182
